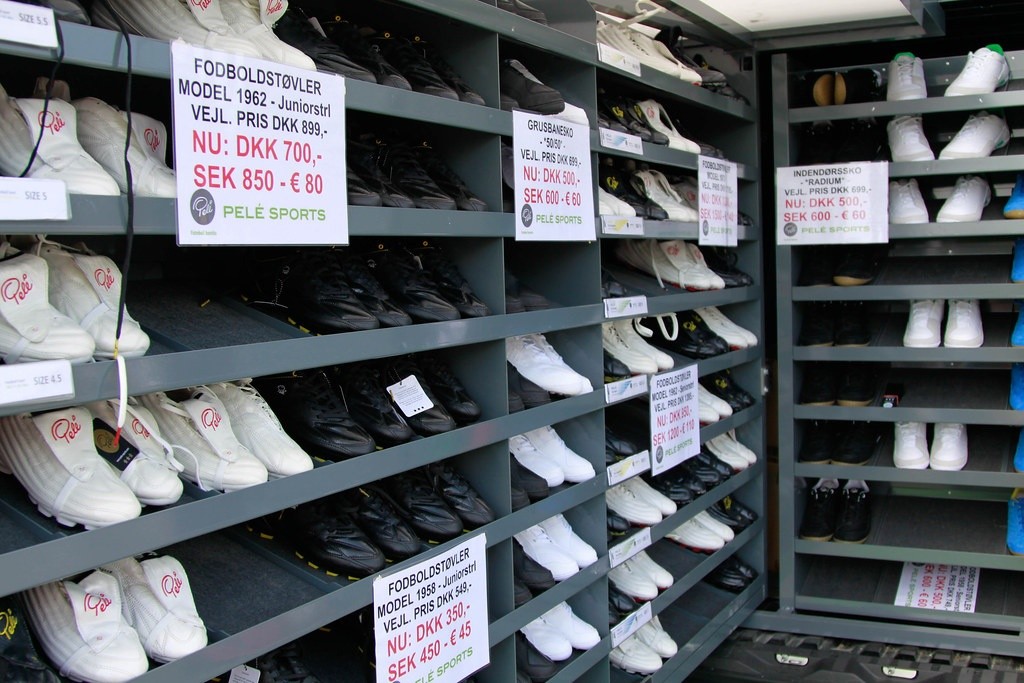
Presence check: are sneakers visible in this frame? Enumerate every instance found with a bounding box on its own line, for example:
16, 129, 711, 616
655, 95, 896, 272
1012, 429, 1024, 473
1003, 177, 1024, 219
903, 299, 943, 348
887, 52, 927, 101
929, 422, 968, 472
1006, 487, 1024, 556
938, 110, 1010, 160
944, 44, 1010, 97
888, 178, 929, 223
936, 175, 991, 223
893, 422, 930, 469
887, 114, 935, 161
0, 0, 880, 683
1009, 363, 1024, 410
944, 298, 984, 348
1010, 240, 1024, 284
1009, 307, 1024, 347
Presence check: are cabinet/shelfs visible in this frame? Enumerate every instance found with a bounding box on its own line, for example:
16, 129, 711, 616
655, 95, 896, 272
0, 0, 1023, 682
